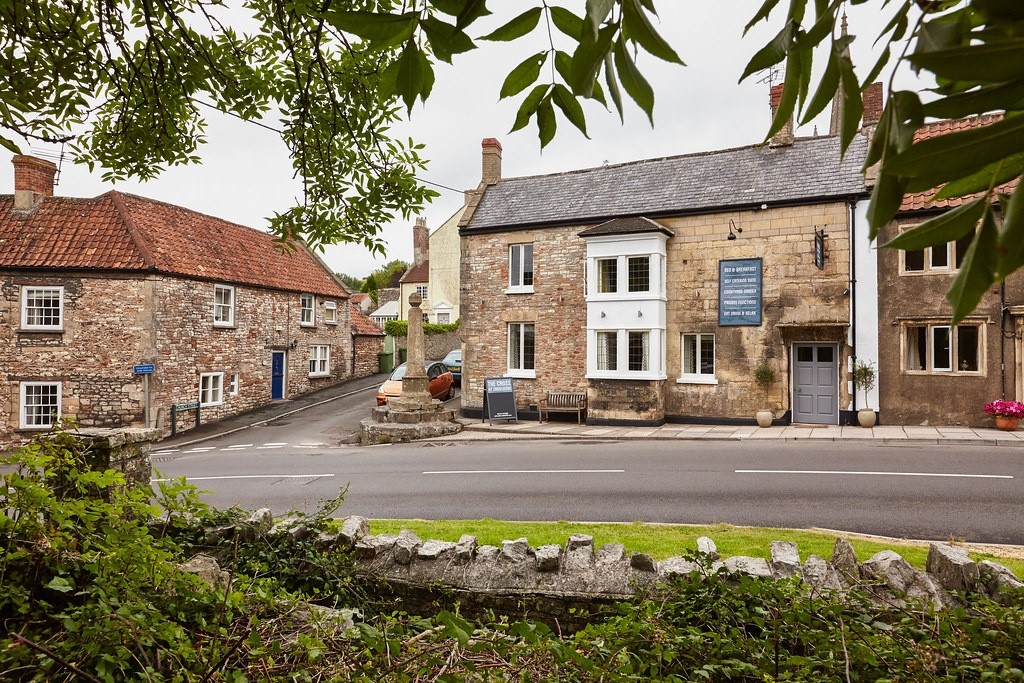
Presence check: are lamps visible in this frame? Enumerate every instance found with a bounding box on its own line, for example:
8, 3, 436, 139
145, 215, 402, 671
727, 219, 742, 241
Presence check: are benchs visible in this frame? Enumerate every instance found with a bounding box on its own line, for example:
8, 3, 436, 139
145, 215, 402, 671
537, 390, 587, 425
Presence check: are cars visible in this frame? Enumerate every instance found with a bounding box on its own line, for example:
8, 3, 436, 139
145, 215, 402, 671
441, 349, 462, 381
375, 360, 455, 408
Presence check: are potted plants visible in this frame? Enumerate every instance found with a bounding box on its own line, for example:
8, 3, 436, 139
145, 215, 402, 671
753, 360, 782, 428
848, 358, 877, 428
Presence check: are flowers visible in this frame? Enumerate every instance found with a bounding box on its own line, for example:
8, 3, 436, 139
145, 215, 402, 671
983, 399, 1024, 421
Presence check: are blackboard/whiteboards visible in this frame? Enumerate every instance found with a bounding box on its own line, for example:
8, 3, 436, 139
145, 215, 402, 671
718, 257, 764, 327
483, 377, 518, 420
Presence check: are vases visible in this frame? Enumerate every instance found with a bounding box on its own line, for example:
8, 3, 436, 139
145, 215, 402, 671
994, 415, 1020, 432
